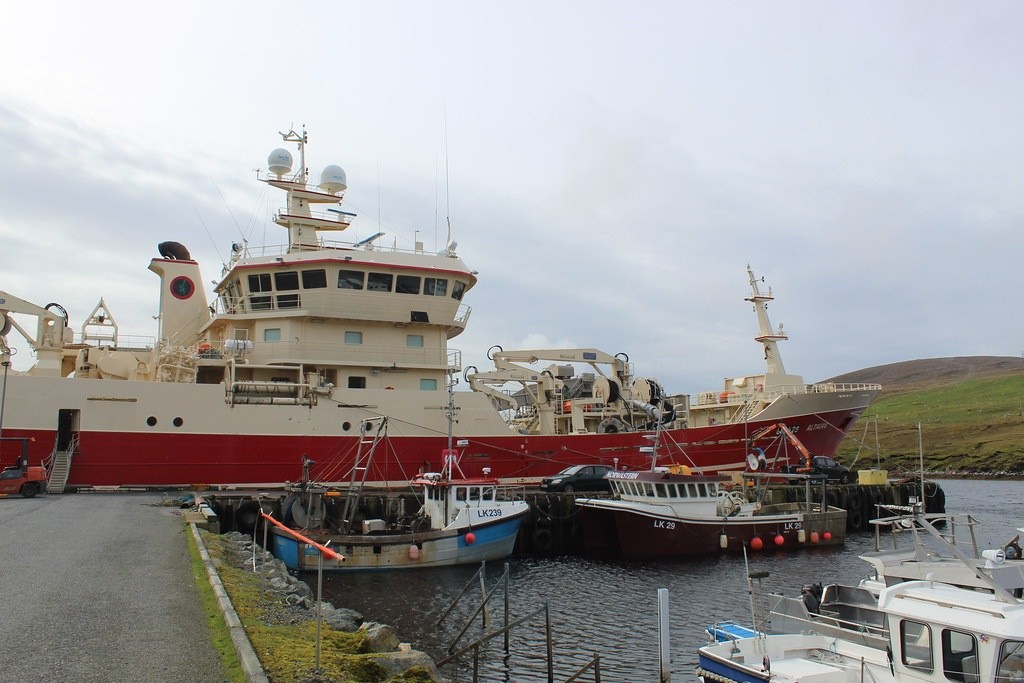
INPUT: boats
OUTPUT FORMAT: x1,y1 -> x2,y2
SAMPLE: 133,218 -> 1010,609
270,365 -> 531,571
0,119 -> 884,492
572,386 -> 848,556
694,420 -> 1024,682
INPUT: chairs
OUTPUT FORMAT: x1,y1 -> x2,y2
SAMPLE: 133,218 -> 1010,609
4,456 -> 21,471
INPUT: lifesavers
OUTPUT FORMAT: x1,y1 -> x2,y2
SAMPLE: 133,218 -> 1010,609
847,492 -> 865,530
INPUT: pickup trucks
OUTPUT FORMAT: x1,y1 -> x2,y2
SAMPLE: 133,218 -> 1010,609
778,455 -> 859,484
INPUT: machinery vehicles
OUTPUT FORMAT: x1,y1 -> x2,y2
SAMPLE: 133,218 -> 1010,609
749,422 -> 828,484
0,435 -> 49,499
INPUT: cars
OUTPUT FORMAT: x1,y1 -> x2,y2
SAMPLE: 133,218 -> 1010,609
539,463 -> 621,493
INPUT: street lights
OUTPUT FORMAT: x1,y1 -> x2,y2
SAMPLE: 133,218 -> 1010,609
0,350 -> 12,429
